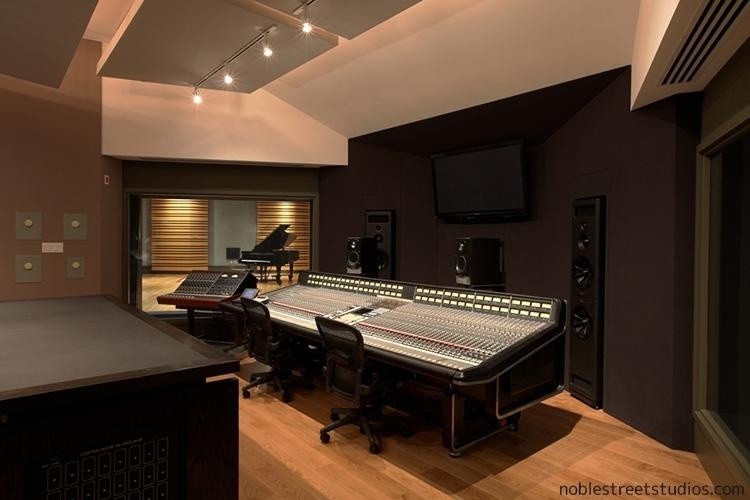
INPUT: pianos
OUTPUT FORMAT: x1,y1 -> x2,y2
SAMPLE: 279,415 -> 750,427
238,225 -> 299,285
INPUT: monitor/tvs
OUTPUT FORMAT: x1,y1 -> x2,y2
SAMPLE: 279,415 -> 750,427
430,137 -> 533,224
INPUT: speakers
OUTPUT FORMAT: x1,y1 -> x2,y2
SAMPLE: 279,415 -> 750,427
455,238 -> 504,286
366,211 -> 395,280
345,236 -> 378,278
569,197 -> 605,410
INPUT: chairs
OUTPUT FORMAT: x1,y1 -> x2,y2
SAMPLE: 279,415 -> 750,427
314,315 -> 415,455
239,296 -> 316,403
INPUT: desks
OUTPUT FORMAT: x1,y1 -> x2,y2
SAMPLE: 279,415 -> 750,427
1,293 -> 241,498
